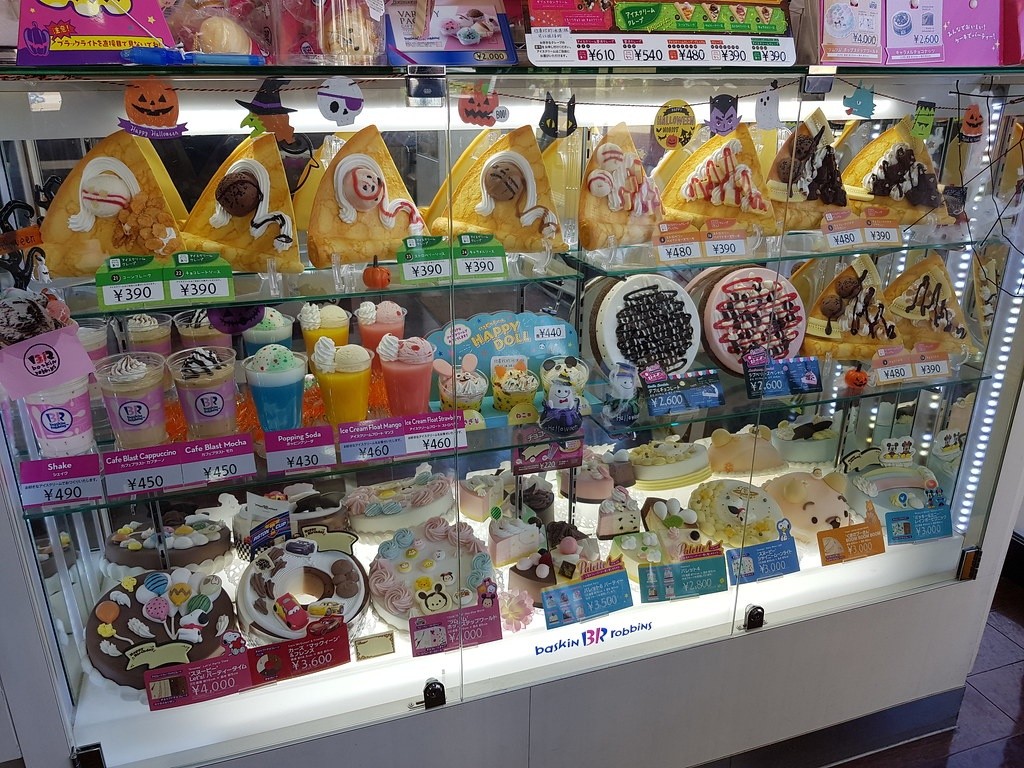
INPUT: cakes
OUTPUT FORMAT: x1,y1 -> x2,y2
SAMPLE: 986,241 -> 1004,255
80,411 -> 947,707
891,11 -> 912,36
696,264 -> 806,378
893,391 -> 976,507
590,274 -> 702,386
823,3 -> 855,38
33,530 -> 77,597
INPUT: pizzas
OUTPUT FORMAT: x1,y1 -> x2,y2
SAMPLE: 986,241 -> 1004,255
674,1 -> 773,25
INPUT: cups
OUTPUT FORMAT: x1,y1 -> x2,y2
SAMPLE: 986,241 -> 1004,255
173,309 -> 233,349
94,351 -> 165,451
354,304 -> 407,369
243,314 -> 295,356
311,347 -> 374,426
126,313 -> 172,357
77,317 -> 108,398
376,339 -> 437,417
298,309 -> 352,372
540,355 -> 589,408
166,346 -> 237,440
491,370 -> 539,411
22,374 -> 94,458
440,365 -> 490,412
240,353 -> 309,432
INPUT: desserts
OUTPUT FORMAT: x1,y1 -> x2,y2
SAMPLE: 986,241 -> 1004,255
30,108 -> 960,286
791,252 -> 979,363
441,9 -> 501,45
0,279 -> 591,464
950,106 -> 1024,351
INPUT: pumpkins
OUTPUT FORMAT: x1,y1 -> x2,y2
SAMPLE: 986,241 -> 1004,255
362,255 -> 392,289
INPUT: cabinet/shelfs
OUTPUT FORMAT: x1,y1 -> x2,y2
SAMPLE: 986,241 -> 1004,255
0,65 -> 1024,768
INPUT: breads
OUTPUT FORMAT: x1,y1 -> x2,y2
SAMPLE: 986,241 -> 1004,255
160,0 -> 377,56
